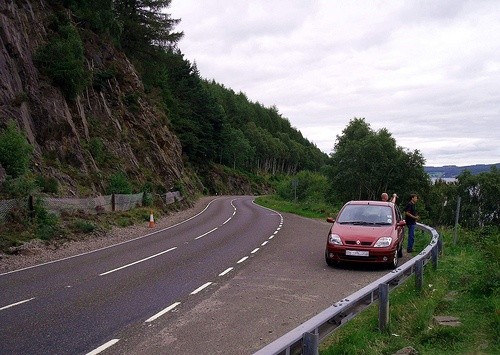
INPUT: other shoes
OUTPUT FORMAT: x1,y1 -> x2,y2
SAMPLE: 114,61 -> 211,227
407,249 -> 417,252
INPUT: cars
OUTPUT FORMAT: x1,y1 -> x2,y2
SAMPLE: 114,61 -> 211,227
325,199 -> 407,270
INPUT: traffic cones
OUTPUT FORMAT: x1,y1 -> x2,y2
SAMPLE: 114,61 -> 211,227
148,209 -> 156,229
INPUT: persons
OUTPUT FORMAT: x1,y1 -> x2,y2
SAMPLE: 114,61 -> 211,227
405,194 -> 420,252
381,193 -> 397,203
